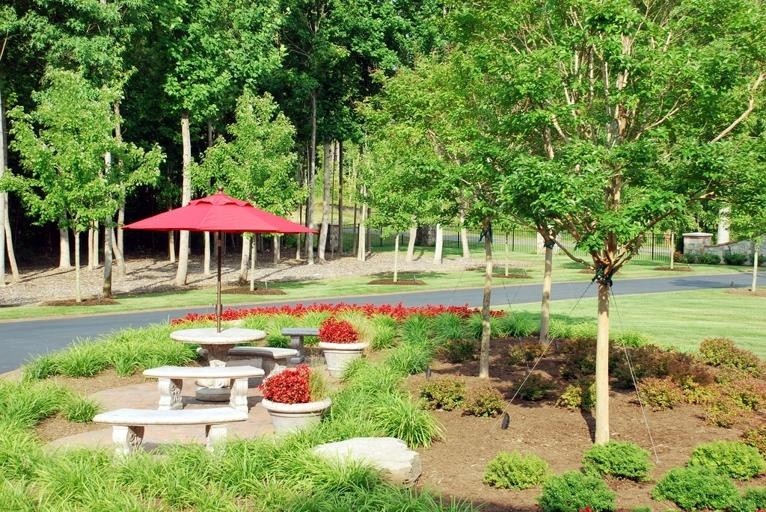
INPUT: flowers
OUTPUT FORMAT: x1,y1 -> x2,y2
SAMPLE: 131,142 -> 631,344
317,314 -> 361,344
255,362 -> 329,403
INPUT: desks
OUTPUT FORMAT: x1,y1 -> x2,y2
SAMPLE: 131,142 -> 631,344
170,325 -> 267,388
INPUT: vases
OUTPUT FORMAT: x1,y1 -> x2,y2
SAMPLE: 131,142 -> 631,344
259,398 -> 330,444
319,341 -> 366,380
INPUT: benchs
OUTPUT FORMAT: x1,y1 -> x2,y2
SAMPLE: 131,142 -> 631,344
88,345 -> 303,458
280,323 -> 320,361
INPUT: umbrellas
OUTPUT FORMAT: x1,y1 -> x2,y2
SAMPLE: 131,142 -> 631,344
122,187 -> 320,332
